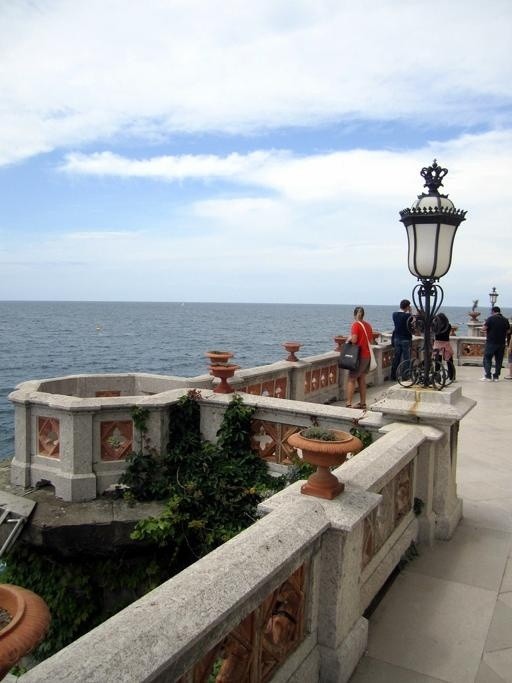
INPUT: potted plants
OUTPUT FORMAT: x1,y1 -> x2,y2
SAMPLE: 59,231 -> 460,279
285,425 -> 364,501
204,350 -> 241,394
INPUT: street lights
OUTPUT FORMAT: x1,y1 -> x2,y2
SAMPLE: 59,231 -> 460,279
488,286 -> 499,307
396,157 -> 468,389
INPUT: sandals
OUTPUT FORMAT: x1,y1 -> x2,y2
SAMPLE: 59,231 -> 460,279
354,403 -> 367,409
346,404 -> 352,408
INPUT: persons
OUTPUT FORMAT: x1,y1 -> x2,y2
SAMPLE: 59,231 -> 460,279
344,306 -> 374,409
429,312 -> 457,381
391,300 -> 413,381
481,306 -> 511,382
503,330 -> 512,380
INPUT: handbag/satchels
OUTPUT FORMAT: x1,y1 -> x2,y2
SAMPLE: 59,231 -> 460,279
368,344 -> 377,372
338,342 -> 361,372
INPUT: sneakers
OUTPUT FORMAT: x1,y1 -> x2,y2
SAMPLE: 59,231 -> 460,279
480,377 -> 499,382
